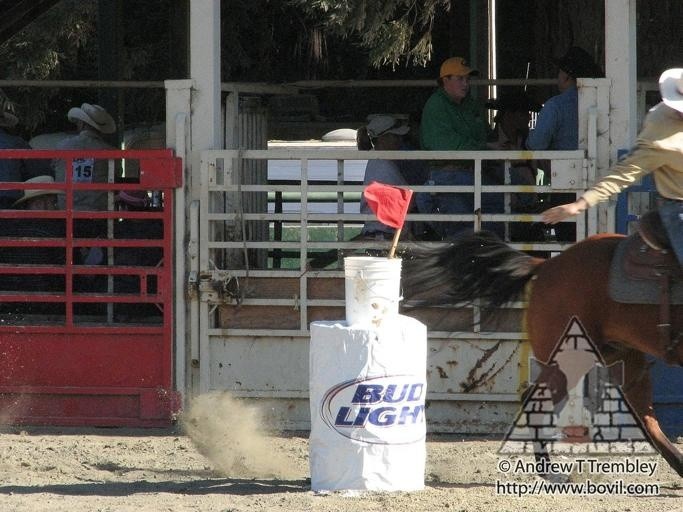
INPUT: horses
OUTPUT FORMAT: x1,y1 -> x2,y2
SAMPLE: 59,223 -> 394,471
400,231 -> 683,483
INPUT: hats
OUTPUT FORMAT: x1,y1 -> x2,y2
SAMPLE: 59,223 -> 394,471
365,114 -> 409,139
11,174 -> 68,208
657,66 -> 683,113
439,56 -> 480,78
67,101 -> 117,136
0,106 -> 21,130
552,46 -> 592,79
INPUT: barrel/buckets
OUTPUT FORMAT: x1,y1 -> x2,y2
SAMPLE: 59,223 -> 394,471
343,255 -> 403,328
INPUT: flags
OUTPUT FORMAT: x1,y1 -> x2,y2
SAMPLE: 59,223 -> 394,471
361,180 -> 412,232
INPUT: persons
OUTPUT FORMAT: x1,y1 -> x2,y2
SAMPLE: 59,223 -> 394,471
354,112 -> 424,238
0,107 -> 53,210
415,57 -> 527,243
76,175 -> 164,322
519,47 -> 606,240
46,101 -> 125,257
480,87 -> 553,226
0,172 -> 67,317
539,66 -> 683,283
